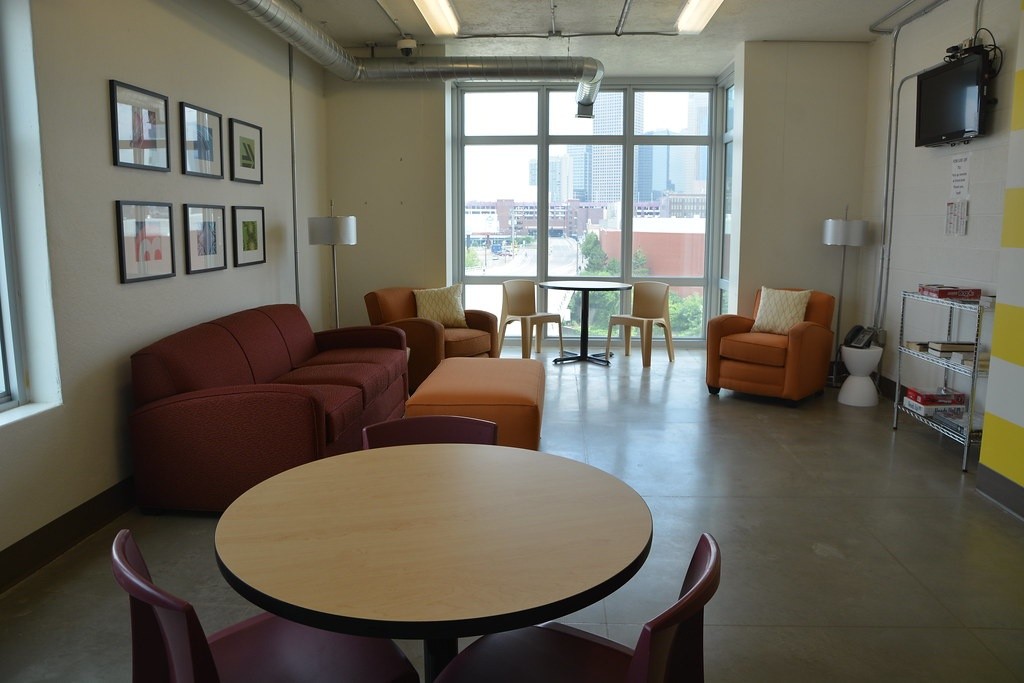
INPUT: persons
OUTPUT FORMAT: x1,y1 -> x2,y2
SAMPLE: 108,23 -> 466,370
126,110 -> 156,151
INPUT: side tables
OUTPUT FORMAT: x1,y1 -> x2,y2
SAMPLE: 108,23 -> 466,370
837,343 -> 883,406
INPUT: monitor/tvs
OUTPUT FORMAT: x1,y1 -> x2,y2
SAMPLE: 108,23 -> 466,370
913,49 -> 991,148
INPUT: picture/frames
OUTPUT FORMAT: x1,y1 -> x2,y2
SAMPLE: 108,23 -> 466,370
183,203 -> 227,274
179,102 -> 225,180
109,78 -> 172,172
230,206 -> 267,267
229,118 -> 263,185
115,200 -> 176,283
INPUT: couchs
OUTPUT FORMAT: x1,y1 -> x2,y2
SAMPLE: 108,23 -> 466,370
362,286 -> 498,396
128,304 -> 407,514
706,286 -> 835,404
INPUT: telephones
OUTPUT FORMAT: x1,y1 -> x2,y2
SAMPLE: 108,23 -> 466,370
844,325 -> 877,349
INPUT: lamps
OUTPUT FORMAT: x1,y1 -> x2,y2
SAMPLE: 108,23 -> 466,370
821,218 -> 869,388
307,200 -> 356,328
414,0 -> 460,40
674,0 -> 724,36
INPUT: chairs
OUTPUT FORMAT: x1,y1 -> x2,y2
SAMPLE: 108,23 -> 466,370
110,525 -> 420,683
605,281 -> 675,368
358,417 -> 498,450
499,278 -> 563,359
442,534 -> 721,683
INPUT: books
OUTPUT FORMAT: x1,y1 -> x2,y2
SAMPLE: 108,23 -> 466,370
905,340 -> 990,369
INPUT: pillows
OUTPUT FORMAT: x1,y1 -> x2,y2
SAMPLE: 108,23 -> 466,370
413,284 -> 467,328
749,288 -> 812,334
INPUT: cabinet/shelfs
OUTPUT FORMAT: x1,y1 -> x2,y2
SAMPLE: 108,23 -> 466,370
893,291 -> 994,475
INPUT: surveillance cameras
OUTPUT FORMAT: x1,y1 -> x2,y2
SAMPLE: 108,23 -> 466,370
396,39 -> 416,57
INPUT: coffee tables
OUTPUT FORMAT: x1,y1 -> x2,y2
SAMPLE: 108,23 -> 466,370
405,357 -> 547,451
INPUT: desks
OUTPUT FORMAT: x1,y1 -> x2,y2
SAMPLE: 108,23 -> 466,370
539,281 -> 633,366
213,442 -> 653,683
